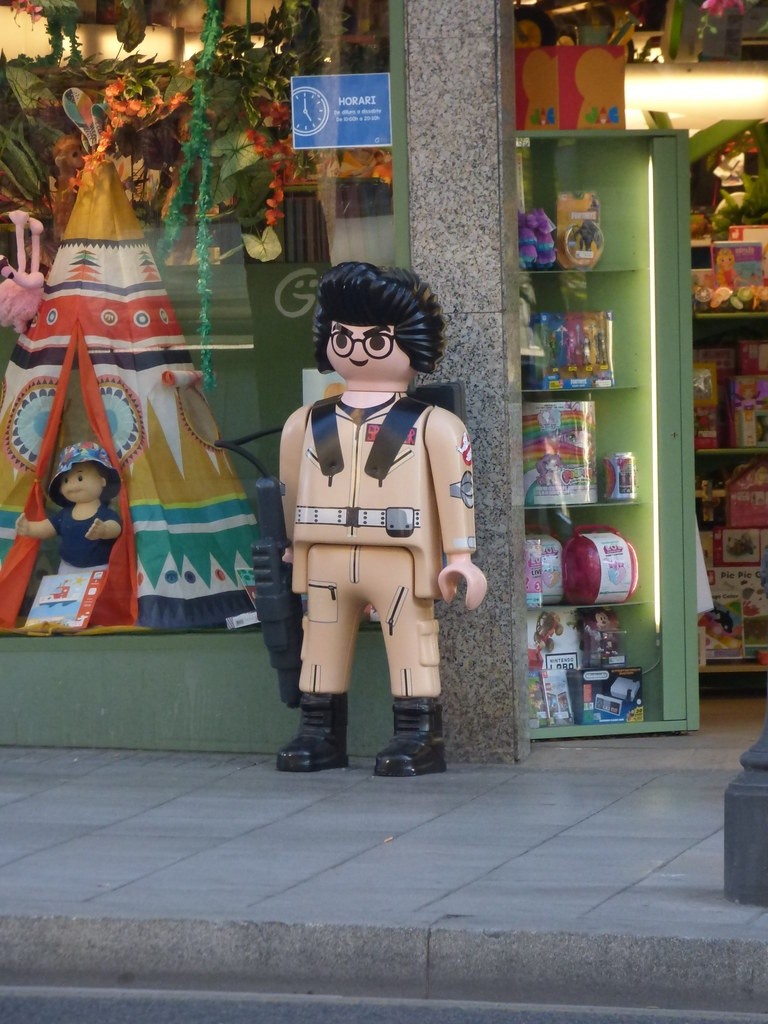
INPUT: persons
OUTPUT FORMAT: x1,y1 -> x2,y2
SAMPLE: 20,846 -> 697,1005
274,263 -> 486,776
14,441 -> 123,574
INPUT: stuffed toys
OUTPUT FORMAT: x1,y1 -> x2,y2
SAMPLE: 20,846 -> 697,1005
0,210 -> 44,334
50,136 -> 86,237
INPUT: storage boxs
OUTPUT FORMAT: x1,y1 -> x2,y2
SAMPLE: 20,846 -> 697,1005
540,668 -> 575,726
527,669 -> 549,728
527,310 -> 617,388
566,666 -> 644,724
692,339 -> 768,660
711,241 -> 761,294
603,630 -> 628,668
729,225 -> 768,286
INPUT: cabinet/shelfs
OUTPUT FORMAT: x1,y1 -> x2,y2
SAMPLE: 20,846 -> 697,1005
692,311 -> 768,672
518,127 -> 702,740
